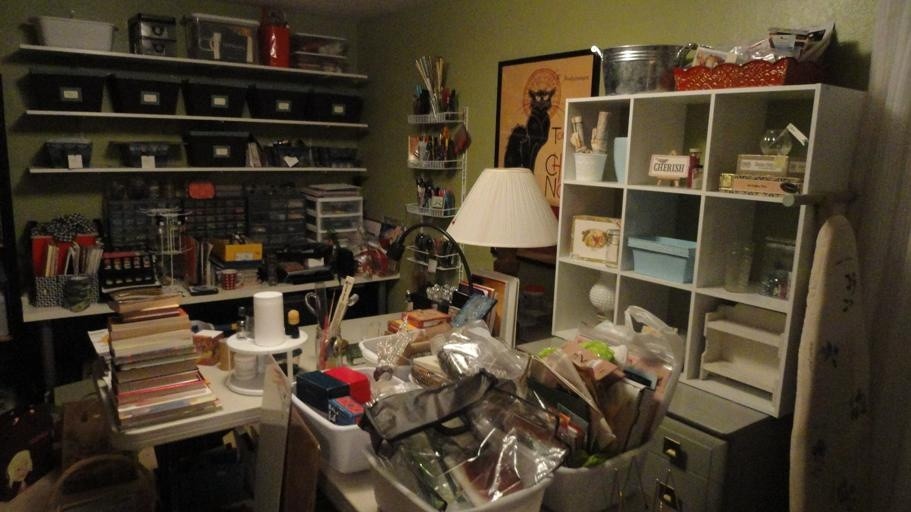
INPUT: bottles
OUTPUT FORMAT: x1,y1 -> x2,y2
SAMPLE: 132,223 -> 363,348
314,326 -> 343,370
687,148 -> 704,190
237,307 -> 254,339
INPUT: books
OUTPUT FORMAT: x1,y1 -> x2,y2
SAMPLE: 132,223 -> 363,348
83,288 -> 226,434
41,242 -> 105,278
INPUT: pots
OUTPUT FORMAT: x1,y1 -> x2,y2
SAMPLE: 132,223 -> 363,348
591,43 -> 699,96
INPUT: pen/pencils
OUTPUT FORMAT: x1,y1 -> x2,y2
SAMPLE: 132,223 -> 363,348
415,234 -> 458,267
444,87 -> 457,120
407,126 -> 455,170
416,178 -> 455,218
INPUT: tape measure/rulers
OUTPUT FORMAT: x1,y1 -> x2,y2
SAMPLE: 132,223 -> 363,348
313,275 -> 356,335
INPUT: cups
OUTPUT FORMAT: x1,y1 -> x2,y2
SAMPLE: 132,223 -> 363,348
221,269 -> 244,291
723,240 -> 755,292
605,229 -> 620,270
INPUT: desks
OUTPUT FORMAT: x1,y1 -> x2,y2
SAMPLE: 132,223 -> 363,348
89,352 -> 291,511
19,267 -> 401,392
273,310 -> 402,511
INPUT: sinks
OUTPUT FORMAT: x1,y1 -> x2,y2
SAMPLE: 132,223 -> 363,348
339,311 -> 406,342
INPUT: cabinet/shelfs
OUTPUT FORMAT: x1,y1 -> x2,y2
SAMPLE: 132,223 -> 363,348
518,335 -> 791,512
103,185 -> 365,252
551,81 -> 868,424
698,302 -> 787,405
407,105 -> 469,286
17,41 -> 371,175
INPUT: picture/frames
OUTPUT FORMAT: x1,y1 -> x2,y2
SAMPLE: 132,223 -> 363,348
494,49 -> 600,209
568,214 -> 621,264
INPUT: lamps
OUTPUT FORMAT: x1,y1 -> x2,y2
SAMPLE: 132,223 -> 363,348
443,166 -> 557,277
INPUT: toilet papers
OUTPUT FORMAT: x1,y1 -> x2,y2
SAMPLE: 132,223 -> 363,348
252,291 -> 285,348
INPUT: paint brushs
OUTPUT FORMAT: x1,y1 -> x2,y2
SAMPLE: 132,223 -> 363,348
415,54 -> 444,123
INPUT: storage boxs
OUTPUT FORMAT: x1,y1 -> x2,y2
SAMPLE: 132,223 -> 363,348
478,404 -> 655,512
360,416 -> 558,512
292,365 -> 416,477
358,331 -> 403,361
292,33 -> 348,74
127,12 -> 260,64
